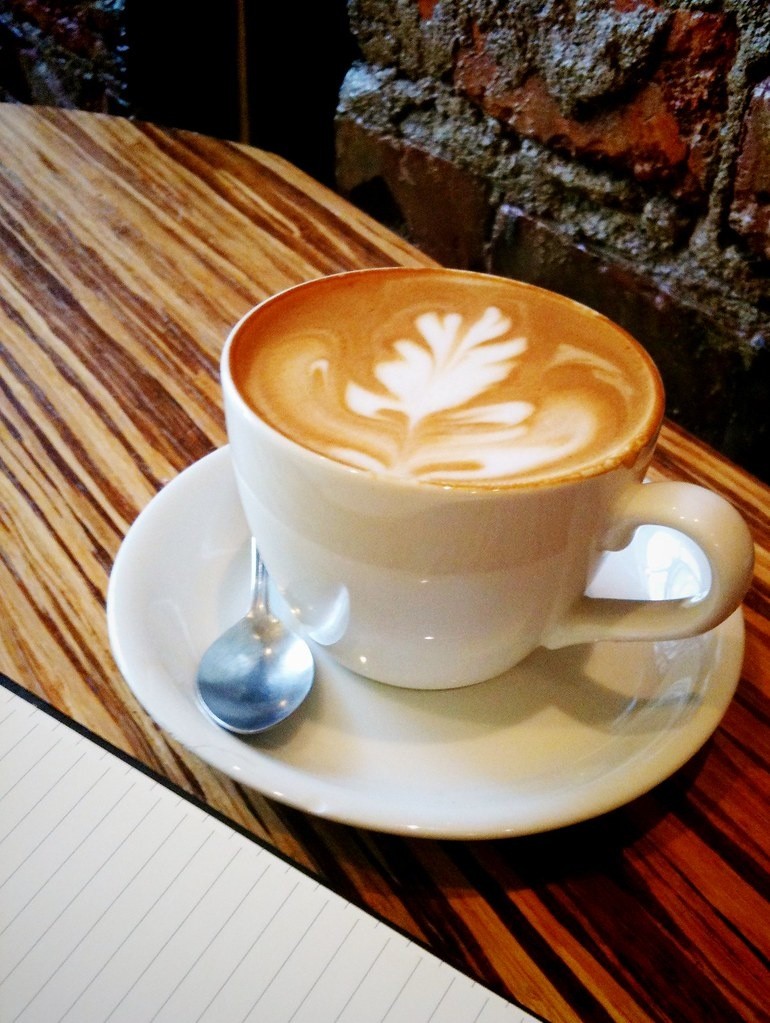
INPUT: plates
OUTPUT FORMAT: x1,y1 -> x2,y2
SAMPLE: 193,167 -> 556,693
106,444 -> 745,840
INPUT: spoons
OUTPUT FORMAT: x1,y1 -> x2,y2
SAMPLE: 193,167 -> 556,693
194,530 -> 313,736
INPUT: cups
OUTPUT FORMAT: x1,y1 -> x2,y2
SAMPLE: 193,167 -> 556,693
220,266 -> 757,690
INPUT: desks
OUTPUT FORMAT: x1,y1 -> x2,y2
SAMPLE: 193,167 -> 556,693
0,104 -> 770,1023
